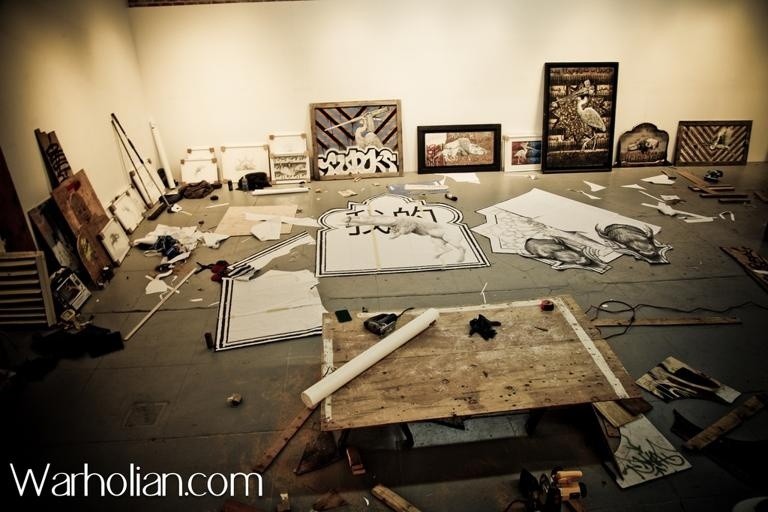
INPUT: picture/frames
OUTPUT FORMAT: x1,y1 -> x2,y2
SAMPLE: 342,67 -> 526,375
269,135 -> 307,156
309,99 -> 403,181
108,190 -> 146,236
221,145 -> 270,182
129,158 -> 167,209
96,216 -> 133,268
180,159 -> 218,185
188,149 -> 215,160
542,61 -> 619,174
115,183 -> 149,211
676,121 -> 753,166
504,136 -> 542,172
269,152 -> 311,185
417,124 -> 501,174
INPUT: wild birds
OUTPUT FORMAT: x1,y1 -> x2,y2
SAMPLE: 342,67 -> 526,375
514,142 -> 538,165
323,106 -> 389,151
567,91 -> 607,156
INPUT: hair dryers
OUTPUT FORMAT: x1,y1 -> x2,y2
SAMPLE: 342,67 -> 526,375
301,309 -> 442,411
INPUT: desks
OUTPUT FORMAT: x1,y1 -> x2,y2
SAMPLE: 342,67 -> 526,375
319,296 -> 645,480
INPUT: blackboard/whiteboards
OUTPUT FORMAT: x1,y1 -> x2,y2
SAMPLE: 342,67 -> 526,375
181,133 -> 309,187
98,159 -> 167,267
